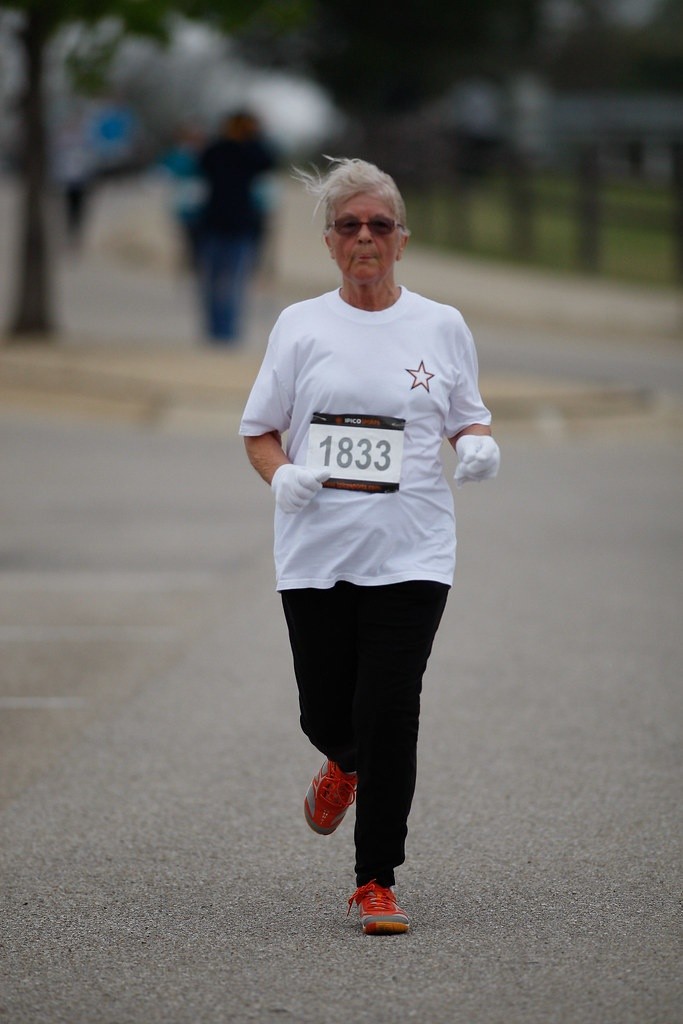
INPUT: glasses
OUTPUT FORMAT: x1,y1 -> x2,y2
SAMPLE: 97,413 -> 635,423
328,215 -> 403,236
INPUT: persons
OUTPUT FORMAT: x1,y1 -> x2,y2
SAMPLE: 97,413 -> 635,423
238,153 -> 500,935
181,110 -> 286,350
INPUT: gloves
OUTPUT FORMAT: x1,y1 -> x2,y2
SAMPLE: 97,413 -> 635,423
270,463 -> 331,516
456,434 -> 499,485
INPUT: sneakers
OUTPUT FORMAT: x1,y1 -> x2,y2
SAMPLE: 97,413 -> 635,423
305,757 -> 357,836
356,884 -> 412,935
